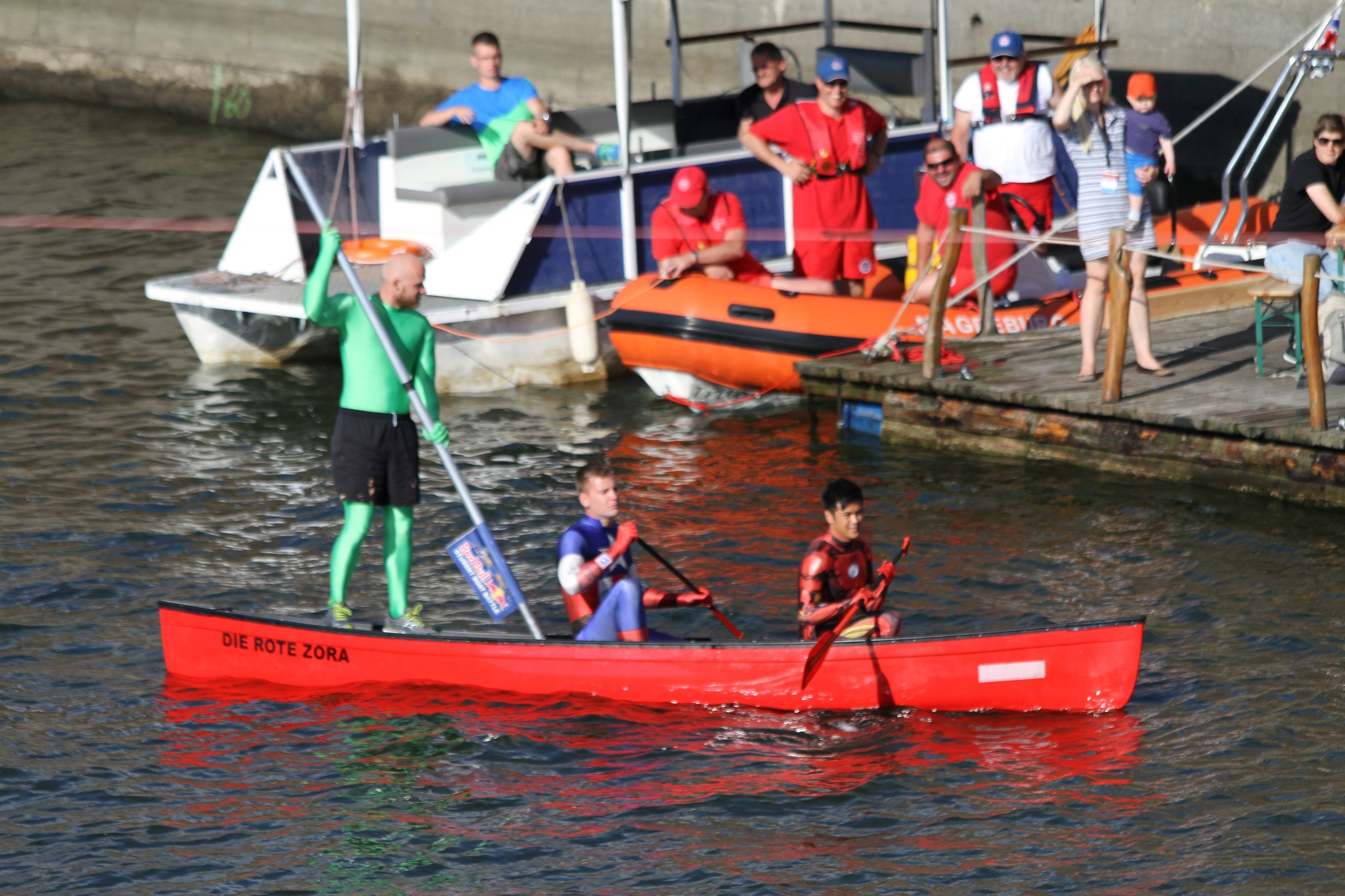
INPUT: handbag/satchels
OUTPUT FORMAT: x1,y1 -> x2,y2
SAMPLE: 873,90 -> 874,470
1150,180 -> 1174,215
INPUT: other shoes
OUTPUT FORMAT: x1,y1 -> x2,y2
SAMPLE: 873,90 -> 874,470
1324,363 -> 1345,385
1283,347 -> 1298,364
598,144 -> 619,167
1122,216 -> 1141,232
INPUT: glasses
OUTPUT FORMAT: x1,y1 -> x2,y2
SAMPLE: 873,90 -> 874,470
1318,137 -> 1345,147
928,157 -> 953,168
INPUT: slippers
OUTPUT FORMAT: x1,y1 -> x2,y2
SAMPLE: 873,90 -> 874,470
1136,363 -> 1172,377
1077,374 -> 1095,383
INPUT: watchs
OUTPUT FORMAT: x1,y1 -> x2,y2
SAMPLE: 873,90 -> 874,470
541,113 -> 552,124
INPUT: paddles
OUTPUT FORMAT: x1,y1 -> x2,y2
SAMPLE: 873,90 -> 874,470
637,535 -> 750,640
801,535 -> 911,689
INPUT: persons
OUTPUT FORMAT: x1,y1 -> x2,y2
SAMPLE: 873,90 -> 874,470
305,219 -> 449,639
651,166 -> 837,295
1122,72 -> 1176,231
420,32 -> 629,180
747,51 -> 887,297
555,463 -> 715,643
1264,114 -> 1345,366
902,138 -> 1019,303
797,477 -> 903,641
950,31 -> 1067,253
1053,56 -> 1173,382
737,42 -> 819,149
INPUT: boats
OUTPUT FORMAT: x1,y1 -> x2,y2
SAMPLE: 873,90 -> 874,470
155,600 -> 1148,714
138,0 -> 1111,399
608,195 -> 1278,414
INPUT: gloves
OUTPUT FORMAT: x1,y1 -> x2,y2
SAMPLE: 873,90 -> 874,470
581,520 -> 637,587
845,590 -> 868,611
641,585 -> 713,609
877,561 -> 896,583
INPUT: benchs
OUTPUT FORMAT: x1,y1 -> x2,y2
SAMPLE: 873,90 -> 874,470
1247,277 -> 1304,381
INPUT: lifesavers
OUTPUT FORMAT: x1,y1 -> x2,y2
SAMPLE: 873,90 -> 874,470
340,238 -> 434,266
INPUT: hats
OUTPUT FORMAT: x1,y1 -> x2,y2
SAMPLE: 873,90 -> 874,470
1127,73 -> 1156,99
817,58 -> 849,83
989,32 -> 1023,58
670,166 -> 707,208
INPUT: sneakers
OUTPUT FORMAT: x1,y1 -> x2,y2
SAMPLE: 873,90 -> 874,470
325,599 -> 354,630
382,602 -> 436,636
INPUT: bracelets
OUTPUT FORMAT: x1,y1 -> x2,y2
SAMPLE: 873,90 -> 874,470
691,250 -> 699,265
